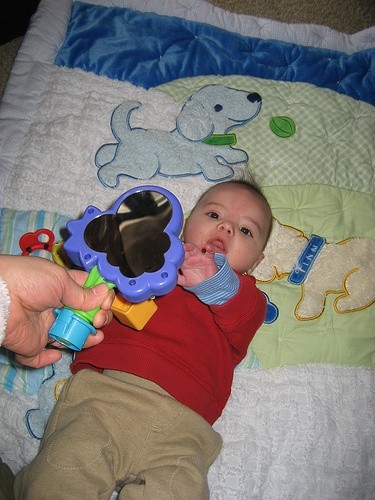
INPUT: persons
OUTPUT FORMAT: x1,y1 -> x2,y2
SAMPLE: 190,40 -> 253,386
1,252 -> 114,369
13,160 -> 272,500
